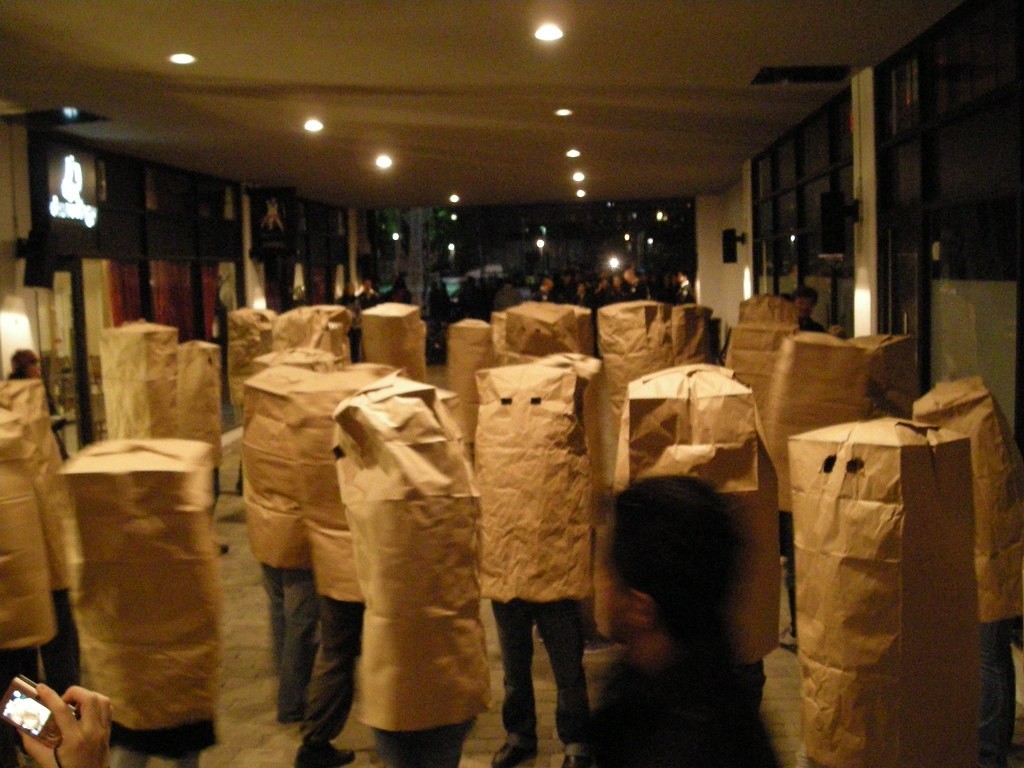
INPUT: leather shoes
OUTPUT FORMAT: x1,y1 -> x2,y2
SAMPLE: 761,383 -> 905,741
491,742 -> 537,768
559,754 -> 586,768
278,711 -> 303,723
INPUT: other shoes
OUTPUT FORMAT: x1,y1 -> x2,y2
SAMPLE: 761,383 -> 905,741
295,742 -> 356,768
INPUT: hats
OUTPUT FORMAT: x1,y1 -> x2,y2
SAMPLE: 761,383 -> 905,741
12,350 -> 40,371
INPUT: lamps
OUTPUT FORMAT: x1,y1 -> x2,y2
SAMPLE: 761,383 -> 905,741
722,228 -> 746,263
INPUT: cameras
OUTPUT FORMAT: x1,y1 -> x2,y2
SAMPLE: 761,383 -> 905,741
0,674 -> 79,749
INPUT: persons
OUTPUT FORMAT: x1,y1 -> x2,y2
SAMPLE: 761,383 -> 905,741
0,267 -> 1024,768
